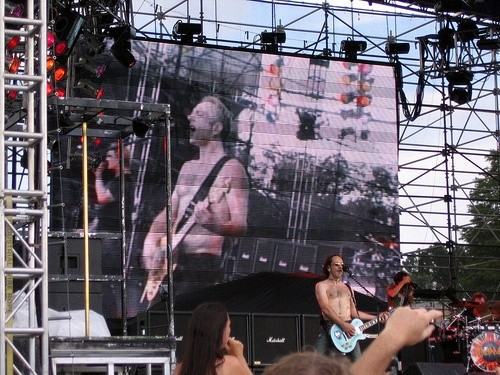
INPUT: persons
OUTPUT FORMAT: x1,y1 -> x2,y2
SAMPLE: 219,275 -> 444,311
262,307 -> 443,375
167,303 -> 252,374
386,271 -> 414,307
312,255 -> 390,362
464,293 -> 487,320
77,96 -> 250,311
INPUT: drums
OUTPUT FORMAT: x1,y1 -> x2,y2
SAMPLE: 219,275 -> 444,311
462,325 -> 495,337
470,332 -> 500,372
439,327 -> 457,342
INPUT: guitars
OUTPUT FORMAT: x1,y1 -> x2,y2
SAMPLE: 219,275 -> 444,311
146,198 -> 211,302
330,311 -> 393,353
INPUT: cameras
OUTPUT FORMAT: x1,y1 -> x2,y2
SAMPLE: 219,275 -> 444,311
404,281 -> 418,289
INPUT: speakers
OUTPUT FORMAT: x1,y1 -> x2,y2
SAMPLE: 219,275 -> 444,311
403,362 -> 467,375
137,310 -> 320,366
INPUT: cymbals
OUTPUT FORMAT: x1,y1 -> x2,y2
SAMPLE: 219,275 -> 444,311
449,300 -> 480,308
478,301 -> 500,319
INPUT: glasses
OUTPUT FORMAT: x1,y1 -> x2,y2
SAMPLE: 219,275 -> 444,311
331,262 -> 345,269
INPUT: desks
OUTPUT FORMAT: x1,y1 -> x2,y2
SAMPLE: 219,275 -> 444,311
50,336 -> 183,375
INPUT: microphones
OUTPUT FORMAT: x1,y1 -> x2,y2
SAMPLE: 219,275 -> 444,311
342,266 -> 354,277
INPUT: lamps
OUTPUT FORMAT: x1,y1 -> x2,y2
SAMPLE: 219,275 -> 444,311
110,45 -> 137,68
5,4 -> 67,99
175,23 -> 202,34
77,52 -> 105,79
385,43 -> 410,54
75,80 -> 106,100
267,58 -> 376,142
259,32 -> 286,44
340,39 -> 366,51
